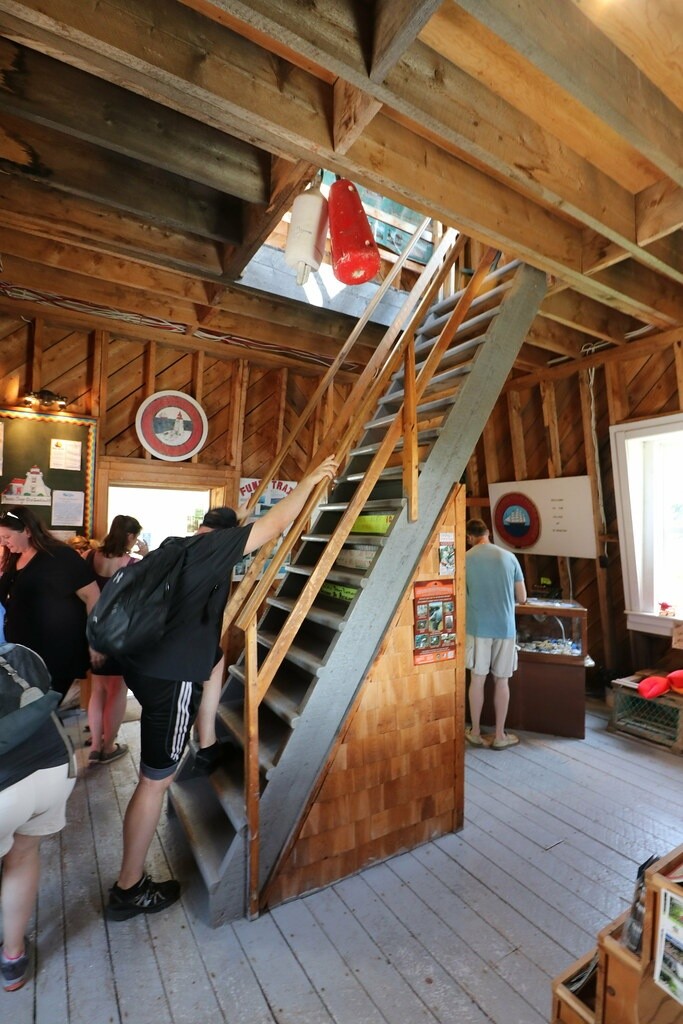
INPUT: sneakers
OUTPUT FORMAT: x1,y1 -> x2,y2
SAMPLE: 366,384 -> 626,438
191,740 -> 236,778
0,936 -> 32,991
104,871 -> 181,922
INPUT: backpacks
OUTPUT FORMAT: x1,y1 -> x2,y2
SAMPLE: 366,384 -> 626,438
81,535 -> 226,666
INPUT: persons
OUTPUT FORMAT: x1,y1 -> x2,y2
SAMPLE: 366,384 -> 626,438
0,454 -> 339,992
466,518 -> 527,750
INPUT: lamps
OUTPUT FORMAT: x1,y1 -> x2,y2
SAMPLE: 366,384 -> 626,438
25,389 -> 67,410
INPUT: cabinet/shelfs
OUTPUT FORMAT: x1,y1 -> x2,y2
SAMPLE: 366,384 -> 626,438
475,599 -> 589,739
552,843 -> 683,1024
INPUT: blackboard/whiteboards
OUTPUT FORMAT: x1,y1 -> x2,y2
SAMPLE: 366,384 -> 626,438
0,404 -> 102,546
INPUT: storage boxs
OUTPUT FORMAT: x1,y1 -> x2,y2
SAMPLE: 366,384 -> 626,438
606,668 -> 683,757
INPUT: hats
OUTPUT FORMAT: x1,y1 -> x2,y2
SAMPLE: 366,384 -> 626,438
201,506 -> 238,533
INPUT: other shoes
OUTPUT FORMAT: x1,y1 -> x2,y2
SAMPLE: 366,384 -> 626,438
463,722 -> 484,747
87,742 -> 129,765
490,730 -> 521,751
